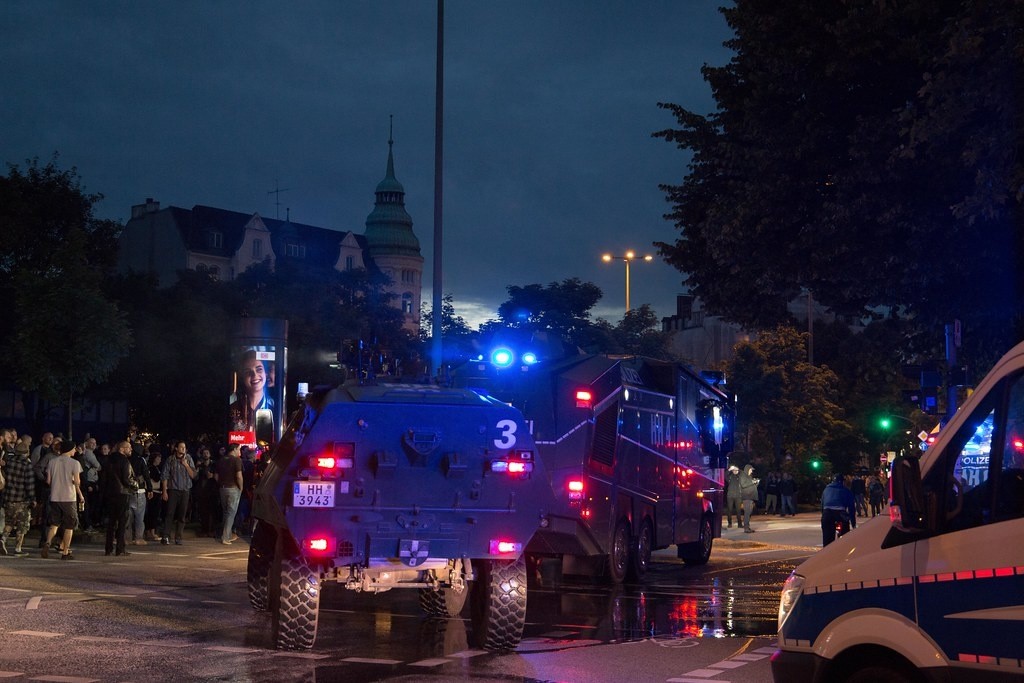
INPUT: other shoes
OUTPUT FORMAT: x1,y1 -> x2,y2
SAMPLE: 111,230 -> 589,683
13,550 -> 29,556
116,551 -> 132,556
105,552 -> 113,556
728,524 -> 732,527
161,536 -> 170,545
84,526 -> 99,533
58,549 -> 72,554
738,525 -> 744,528
148,535 -> 162,540
41,543 -> 50,559
124,539 -> 134,545
231,533 -> 238,541
135,539 -> 147,545
0,540 -> 7,555
61,553 -> 73,560
744,528 -> 754,533
176,538 -> 183,545
223,540 -> 231,544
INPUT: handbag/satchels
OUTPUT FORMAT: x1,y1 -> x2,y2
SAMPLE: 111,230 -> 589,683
144,466 -> 161,490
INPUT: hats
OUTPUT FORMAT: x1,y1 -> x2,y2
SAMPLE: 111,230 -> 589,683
15,442 -> 28,455
60,440 -> 75,453
834,473 -> 846,481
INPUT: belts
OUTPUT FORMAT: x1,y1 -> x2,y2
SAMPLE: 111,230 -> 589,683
221,485 -> 238,489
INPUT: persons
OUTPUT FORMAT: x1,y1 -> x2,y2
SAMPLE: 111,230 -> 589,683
232,350 -> 276,428
725,456 -> 890,547
0,425 -> 274,560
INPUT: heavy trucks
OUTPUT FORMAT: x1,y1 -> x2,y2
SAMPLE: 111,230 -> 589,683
245,346 -> 742,656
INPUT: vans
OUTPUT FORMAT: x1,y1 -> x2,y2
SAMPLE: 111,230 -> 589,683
767,342 -> 1024,683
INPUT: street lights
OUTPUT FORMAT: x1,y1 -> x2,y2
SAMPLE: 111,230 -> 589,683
602,249 -> 654,317
